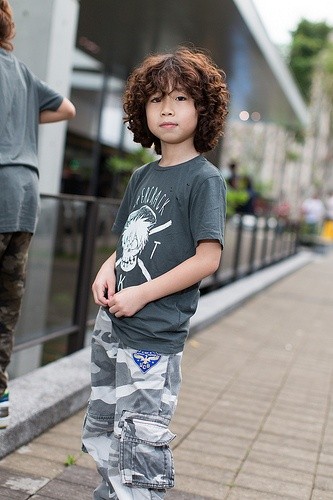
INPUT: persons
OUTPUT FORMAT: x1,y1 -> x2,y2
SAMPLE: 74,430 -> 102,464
222,160 -> 333,232
77,46 -> 231,500
0,1 -> 77,432
